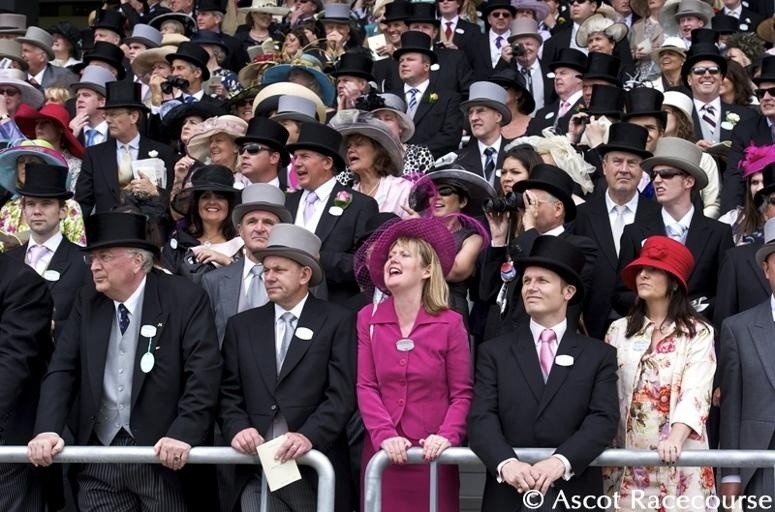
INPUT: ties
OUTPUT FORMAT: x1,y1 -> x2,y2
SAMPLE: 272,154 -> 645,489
118,304 -> 130,335
445,22 -> 452,40
614,204 -> 628,254
26,243 -> 47,273
273,313 -> 296,444
540,328 -> 556,376
245,267 -> 266,309
670,222 -> 686,245
300,194 -> 318,228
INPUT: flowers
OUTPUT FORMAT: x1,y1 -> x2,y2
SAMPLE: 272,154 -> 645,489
332,188 -> 355,213
724,113 -> 742,129
420,91 -> 440,106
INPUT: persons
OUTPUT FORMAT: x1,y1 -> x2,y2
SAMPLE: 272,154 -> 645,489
0,0 -> 775,511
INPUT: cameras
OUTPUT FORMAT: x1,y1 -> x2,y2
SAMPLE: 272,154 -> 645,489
511,43 -> 527,56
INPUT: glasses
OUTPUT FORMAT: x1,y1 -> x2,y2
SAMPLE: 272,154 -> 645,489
427,187 -> 463,198
650,169 -> 684,178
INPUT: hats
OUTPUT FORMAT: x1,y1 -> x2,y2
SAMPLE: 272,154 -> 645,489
597,122 -> 654,165
251,223 -> 323,287
737,143 -> 775,270
512,236 -> 587,303
620,234 -> 696,295
409,163 -> 497,216
0,0 -> 441,174
171,164 -> 241,216
458,0 -> 775,129
15,162 -> 74,200
640,136 -> 708,190
367,217 -> 457,296
231,184 -> 293,227
512,163 -> 577,223
77,212 -> 160,256
